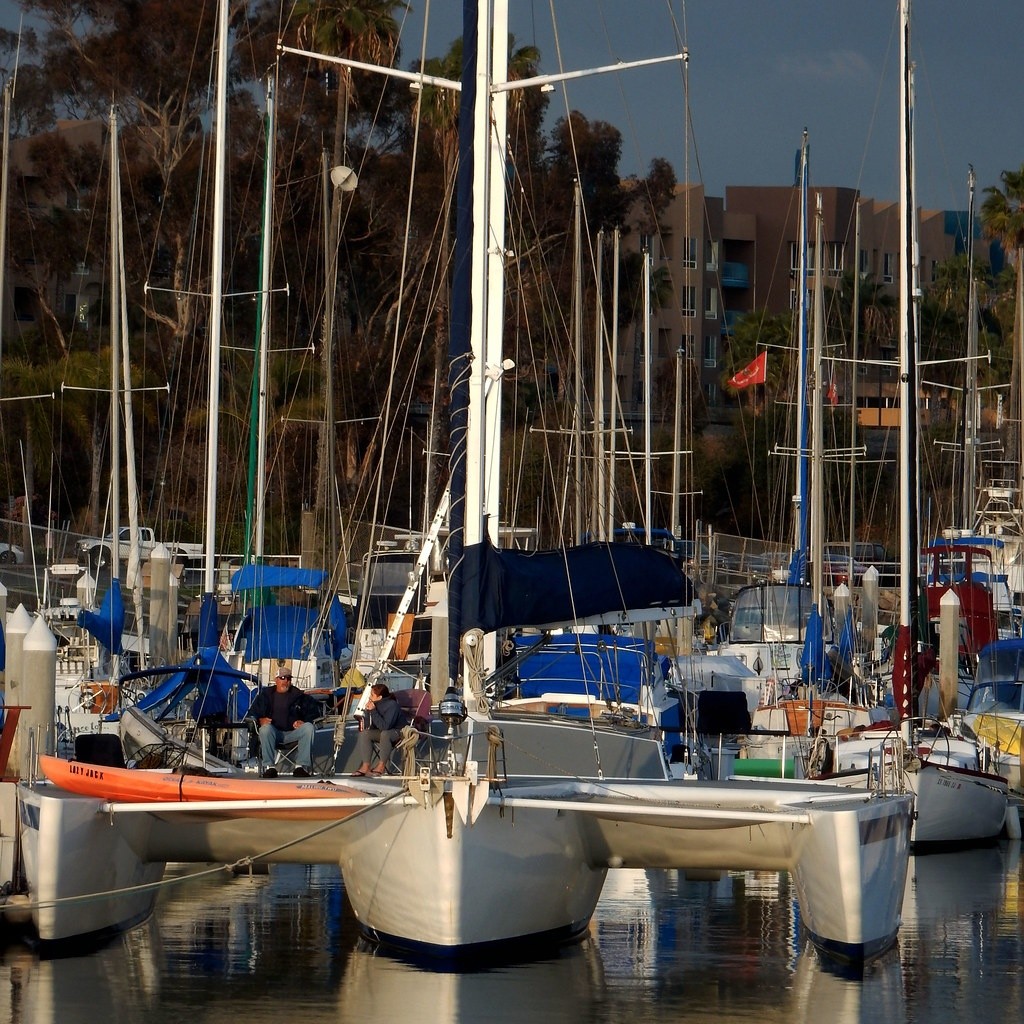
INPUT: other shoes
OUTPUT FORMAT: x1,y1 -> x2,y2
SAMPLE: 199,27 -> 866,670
293,767 -> 310,777
263,768 -> 278,778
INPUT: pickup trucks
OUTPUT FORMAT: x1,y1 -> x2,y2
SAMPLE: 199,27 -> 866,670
75,525 -> 204,571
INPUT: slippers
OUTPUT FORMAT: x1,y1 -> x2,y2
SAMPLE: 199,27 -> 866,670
366,768 -> 385,777
351,770 -> 370,777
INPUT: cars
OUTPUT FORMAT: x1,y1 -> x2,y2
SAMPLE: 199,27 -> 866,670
809,554 -> 870,586
719,552 -> 771,582
0,543 -> 26,565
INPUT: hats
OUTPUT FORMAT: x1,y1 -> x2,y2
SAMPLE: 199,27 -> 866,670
276,668 -> 293,678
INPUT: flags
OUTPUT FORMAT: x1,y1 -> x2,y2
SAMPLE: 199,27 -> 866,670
726,350 -> 766,387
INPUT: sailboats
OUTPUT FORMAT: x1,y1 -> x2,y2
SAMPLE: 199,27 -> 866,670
0,0 -> 1024,966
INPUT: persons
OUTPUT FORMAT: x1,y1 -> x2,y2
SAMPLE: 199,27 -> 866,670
351,684 -> 406,777
249,667 -> 316,778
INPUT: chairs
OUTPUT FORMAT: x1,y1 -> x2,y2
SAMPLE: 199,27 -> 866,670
370,689 -> 439,773
247,716 -> 314,777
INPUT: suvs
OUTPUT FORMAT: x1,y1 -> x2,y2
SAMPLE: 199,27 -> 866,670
674,540 -> 727,581
811,543 -> 888,567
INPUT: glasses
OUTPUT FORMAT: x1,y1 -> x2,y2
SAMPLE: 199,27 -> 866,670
278,676 -> 291,680
